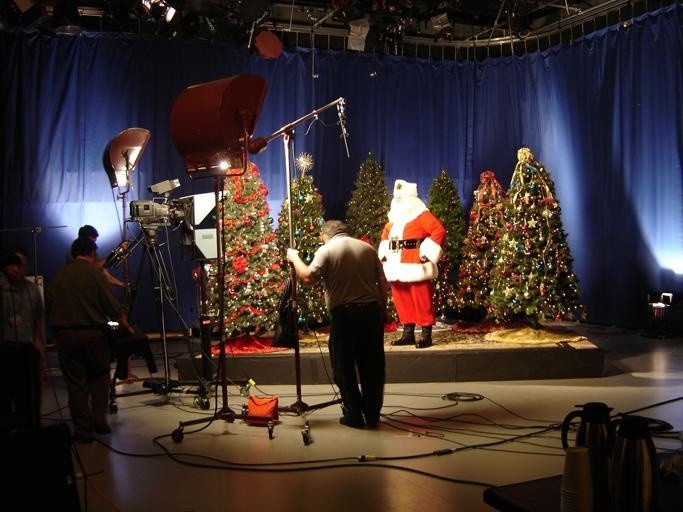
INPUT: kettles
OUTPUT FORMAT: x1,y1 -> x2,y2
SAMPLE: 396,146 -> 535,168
561,401 -> 659,512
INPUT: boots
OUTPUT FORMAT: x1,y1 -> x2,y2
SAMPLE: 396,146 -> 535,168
416,326 -> 432,348
391,324 -> 415,345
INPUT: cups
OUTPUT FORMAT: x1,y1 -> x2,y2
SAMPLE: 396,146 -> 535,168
560,447 -> 592,512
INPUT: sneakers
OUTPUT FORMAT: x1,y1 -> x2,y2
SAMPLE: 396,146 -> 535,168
340,418 -> 377,428
91,416 -> 110,434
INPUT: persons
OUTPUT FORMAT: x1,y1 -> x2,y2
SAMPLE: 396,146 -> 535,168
286,219 -> 389,430
0,246 -> 44,435
66,225 -> 131,288
43,237 -> 136,444
379,177 -> 447,348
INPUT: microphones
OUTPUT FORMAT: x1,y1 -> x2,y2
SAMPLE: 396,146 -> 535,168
338,119 -> 351,159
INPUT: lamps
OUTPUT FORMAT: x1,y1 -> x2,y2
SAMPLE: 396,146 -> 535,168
142,0 -> 176,23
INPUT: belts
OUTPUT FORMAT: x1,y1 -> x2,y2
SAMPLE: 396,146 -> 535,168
389,240 -> 423,250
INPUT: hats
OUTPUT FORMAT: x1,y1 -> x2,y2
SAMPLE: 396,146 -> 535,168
394,179 -> 417,196
